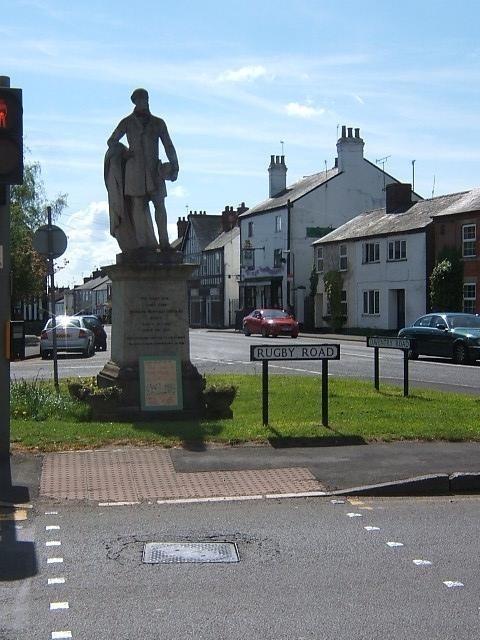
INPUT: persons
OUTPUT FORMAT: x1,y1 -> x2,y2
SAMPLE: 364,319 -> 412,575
105,88 -> 180,256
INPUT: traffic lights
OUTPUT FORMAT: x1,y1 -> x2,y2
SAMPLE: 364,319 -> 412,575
0,88 -> 23,186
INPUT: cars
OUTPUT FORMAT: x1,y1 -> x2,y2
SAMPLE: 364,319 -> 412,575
397,311 -> 480,365
38,314 -> 97,359
75,314 -> 109,351
241,307 -> 302,339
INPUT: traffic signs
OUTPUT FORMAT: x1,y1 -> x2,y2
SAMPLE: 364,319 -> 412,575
249,343 -> 340,361
366,336 -> 417,349
